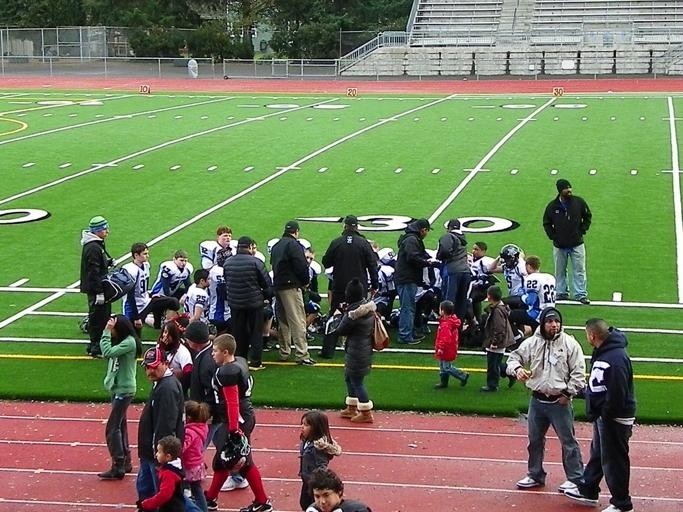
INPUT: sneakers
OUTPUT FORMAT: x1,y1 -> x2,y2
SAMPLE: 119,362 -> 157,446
517,478 -> 540,488
248,334 -> 315,371
581,298 -> 590,303
555,294 -> 568,299
482,386 -> 498,391
240,500 -> 273,511
601,503 -> 634,512
98,470 -> 125,480
508,375 -> 516,387
399,325 -> 430,345
558,481 -> 598,506
435,384 -> 447,387
220,475 -> 249,491
460,374 -> 469,386
206,500 -> 218,509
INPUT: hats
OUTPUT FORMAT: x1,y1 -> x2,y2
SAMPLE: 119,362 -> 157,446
285,222 -> 299,233
544,310 -> 561,323
345,278 -> 363,302
238,238 -> 252,247
448,219 -> 460,228
416,219 -> 430,231
89,216 -> 108,232
140,347 -> 165,367
557,180 -> 571,192
343,214 -> 356,226
185,321 -> 209,344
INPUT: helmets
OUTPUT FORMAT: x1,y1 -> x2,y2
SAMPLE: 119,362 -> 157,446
221,431 -> 252,473
501,244 -> 520,269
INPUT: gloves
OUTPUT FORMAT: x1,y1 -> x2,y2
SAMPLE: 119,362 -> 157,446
95,294 -> 105,305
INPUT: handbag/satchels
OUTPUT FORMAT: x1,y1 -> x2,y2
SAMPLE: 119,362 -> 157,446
373,318 -> 390,350
100,268 -> 135,303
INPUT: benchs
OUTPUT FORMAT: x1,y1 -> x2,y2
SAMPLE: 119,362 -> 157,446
408,1 -> 670,48
342,47 -> 682,75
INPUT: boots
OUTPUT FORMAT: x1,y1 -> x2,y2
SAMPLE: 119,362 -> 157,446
340,396 -> 358,417
351,400 -> 374,423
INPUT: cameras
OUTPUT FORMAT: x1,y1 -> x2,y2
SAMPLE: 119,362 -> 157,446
338,302 -> 349,313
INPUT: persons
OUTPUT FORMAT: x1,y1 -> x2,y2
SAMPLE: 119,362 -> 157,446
506,307 -> 586,492
564,318 -> 635,512
543,179 -> 592,305
